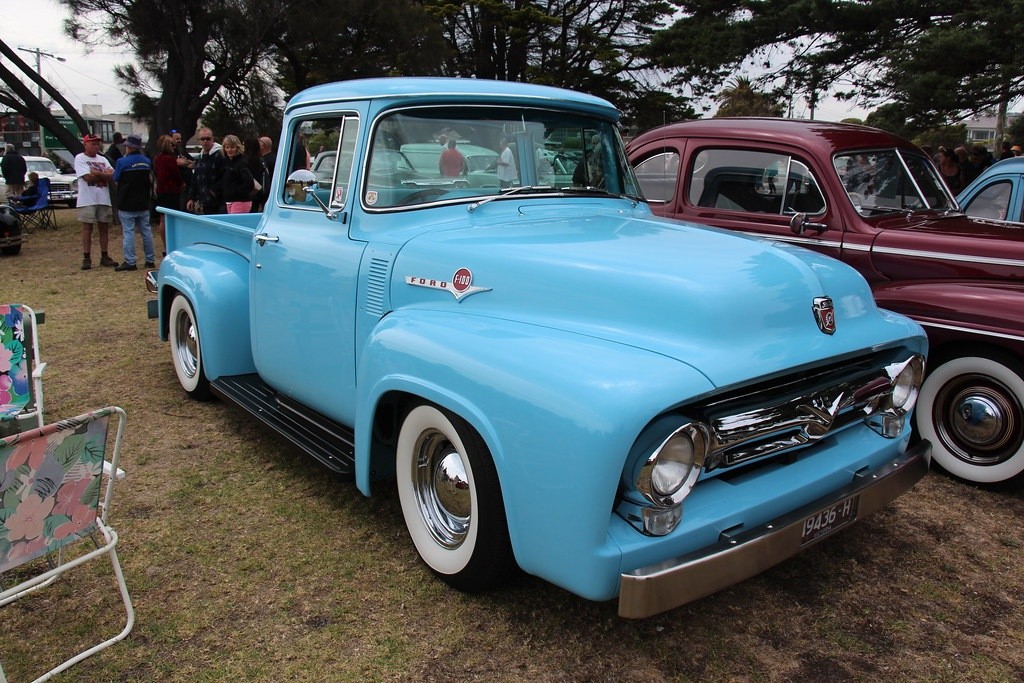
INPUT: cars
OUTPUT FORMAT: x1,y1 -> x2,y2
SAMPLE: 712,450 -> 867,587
394,143 -> 581,190
0,156 -> 80,209
622,118 -> 1024,494
950,153 -> 1024,222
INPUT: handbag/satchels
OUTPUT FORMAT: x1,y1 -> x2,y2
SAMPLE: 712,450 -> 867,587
248,178 -> 262,197
226,201 -> 252,213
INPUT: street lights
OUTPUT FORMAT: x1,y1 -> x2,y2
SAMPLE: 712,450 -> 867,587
35,49 -> 69,156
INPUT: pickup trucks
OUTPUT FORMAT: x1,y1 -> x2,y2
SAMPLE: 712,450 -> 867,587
144,77 -> 932,621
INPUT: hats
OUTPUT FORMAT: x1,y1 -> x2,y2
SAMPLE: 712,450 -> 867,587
7,143 -> 15,151
114,132 -> 126,145
123,135 -> 145,150
1011,145 -> 1023,154
83,134 -> 103,145
971,146 -> 993,154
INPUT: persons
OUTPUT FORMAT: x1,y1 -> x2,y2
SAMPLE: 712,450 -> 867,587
754,142 -> 1024,206
0,144 -> 40,227
74,134 -> 119,270
438,134 -> 604,189
105,127 -> 327,271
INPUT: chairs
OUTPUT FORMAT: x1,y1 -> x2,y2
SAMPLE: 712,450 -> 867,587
9,178 -> 57,233
0,304 -> 135,683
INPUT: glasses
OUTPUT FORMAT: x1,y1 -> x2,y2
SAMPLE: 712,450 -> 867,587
170,129 -> 177,133
200,137 -> 213,141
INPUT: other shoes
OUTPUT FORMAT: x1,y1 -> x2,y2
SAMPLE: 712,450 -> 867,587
82,258 -> 91,269
100,257 -> 119,267
115,262 -> 138,271
145,263 -> 155,268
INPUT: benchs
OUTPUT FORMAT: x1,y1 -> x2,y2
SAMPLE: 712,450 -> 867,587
638,176 -> 741,204
309,188 -> 450,206
